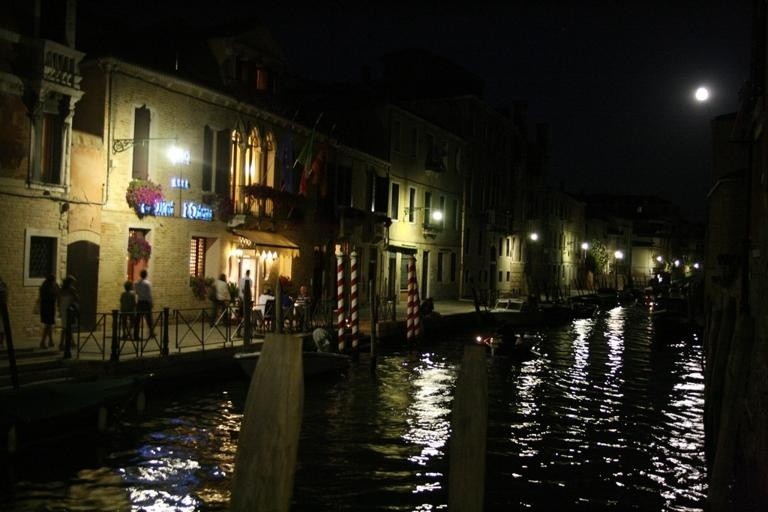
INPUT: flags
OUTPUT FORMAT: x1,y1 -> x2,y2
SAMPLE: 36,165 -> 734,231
294,127 -> 316,167
298,149 -> 322,196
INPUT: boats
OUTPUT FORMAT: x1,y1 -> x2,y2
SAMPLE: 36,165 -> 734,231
574,282 -> 684,317
232,349 -> 353,383
489,297 -> 573,325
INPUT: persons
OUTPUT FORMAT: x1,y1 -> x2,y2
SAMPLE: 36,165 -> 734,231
282,289 -> 293,309
207,273 -> 232,327
56,274 -> 81,350
37,273 -> 60,348
257,287 -> 276,320
239,269 -> 253,313
134,269 -> 158,341
118,279 -> 137,339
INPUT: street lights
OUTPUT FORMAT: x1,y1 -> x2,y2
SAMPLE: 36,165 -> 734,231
613,249 -> 623,290
